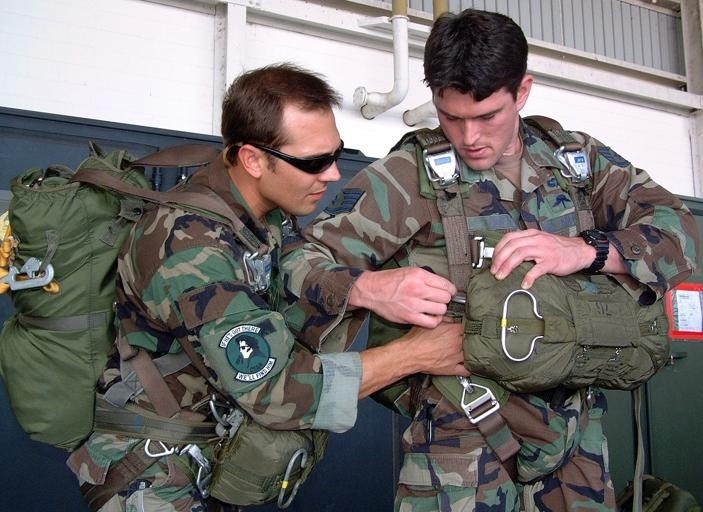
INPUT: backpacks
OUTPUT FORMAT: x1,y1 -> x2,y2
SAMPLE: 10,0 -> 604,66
0,141 -> 268,448
365,116 -> 596,417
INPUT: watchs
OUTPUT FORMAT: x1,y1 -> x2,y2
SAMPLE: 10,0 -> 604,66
576,229 -> 609,274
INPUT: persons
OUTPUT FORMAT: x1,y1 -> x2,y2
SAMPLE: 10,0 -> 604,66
66,64 -> 470,511
279,10 -> 699,512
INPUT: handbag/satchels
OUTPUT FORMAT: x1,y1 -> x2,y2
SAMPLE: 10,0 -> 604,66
463,259 -> 670,394
209,415 -> 330,505
616,474 -> 702,511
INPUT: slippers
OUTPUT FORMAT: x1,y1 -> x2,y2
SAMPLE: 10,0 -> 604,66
244,139 -> 344,174
